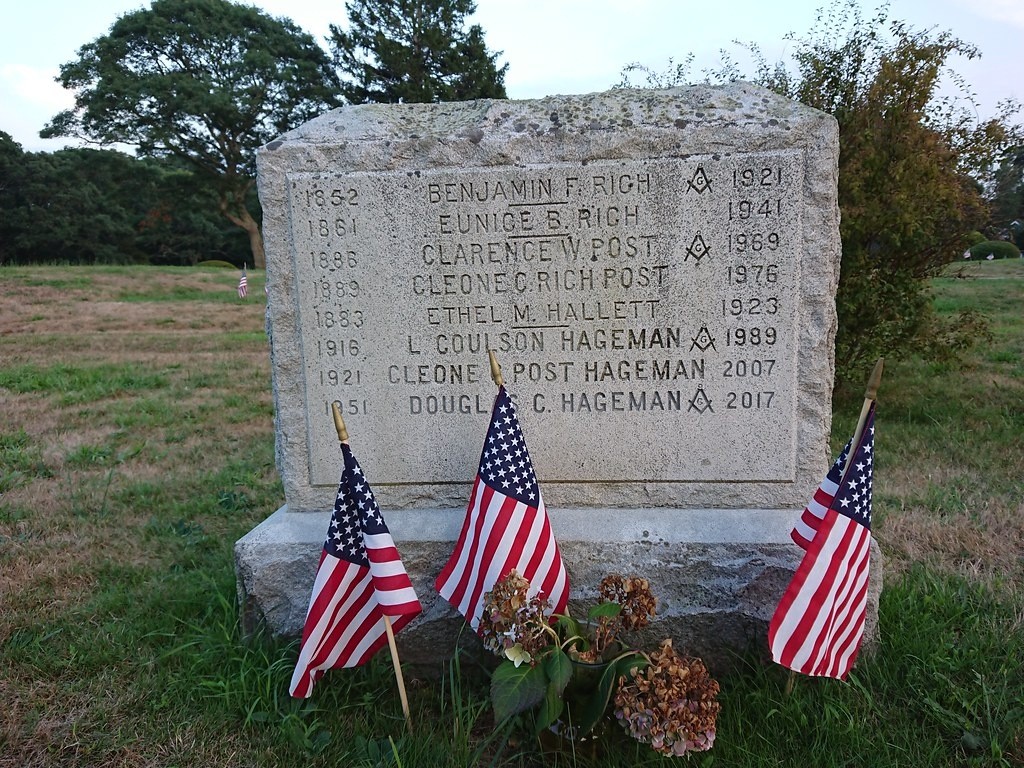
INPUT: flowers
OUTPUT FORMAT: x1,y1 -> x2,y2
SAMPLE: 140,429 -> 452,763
472,567 -> 722,757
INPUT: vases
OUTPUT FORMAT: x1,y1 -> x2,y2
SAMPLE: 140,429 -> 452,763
549,621 -> 623,721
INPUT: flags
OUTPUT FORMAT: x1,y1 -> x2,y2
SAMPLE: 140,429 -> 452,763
987,254 -> 994,260
238,269 -> 248,298
288,443 -> 423,698
964,248 -> 971,259
768,399 -> 875,680
434,384 -> 570,639
